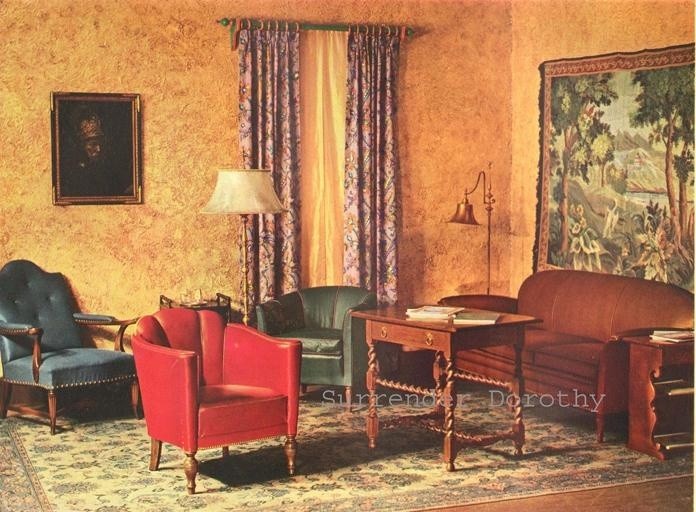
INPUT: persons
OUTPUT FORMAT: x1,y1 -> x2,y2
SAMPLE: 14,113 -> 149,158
59,113 -> 133,196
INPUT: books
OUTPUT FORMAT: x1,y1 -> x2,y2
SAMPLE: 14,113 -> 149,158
407,308 -> 456,319
453,312 -> 501,324
405,305 -> 465,316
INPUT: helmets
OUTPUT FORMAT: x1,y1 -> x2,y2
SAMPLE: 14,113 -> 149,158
79,119 -> 105,139
